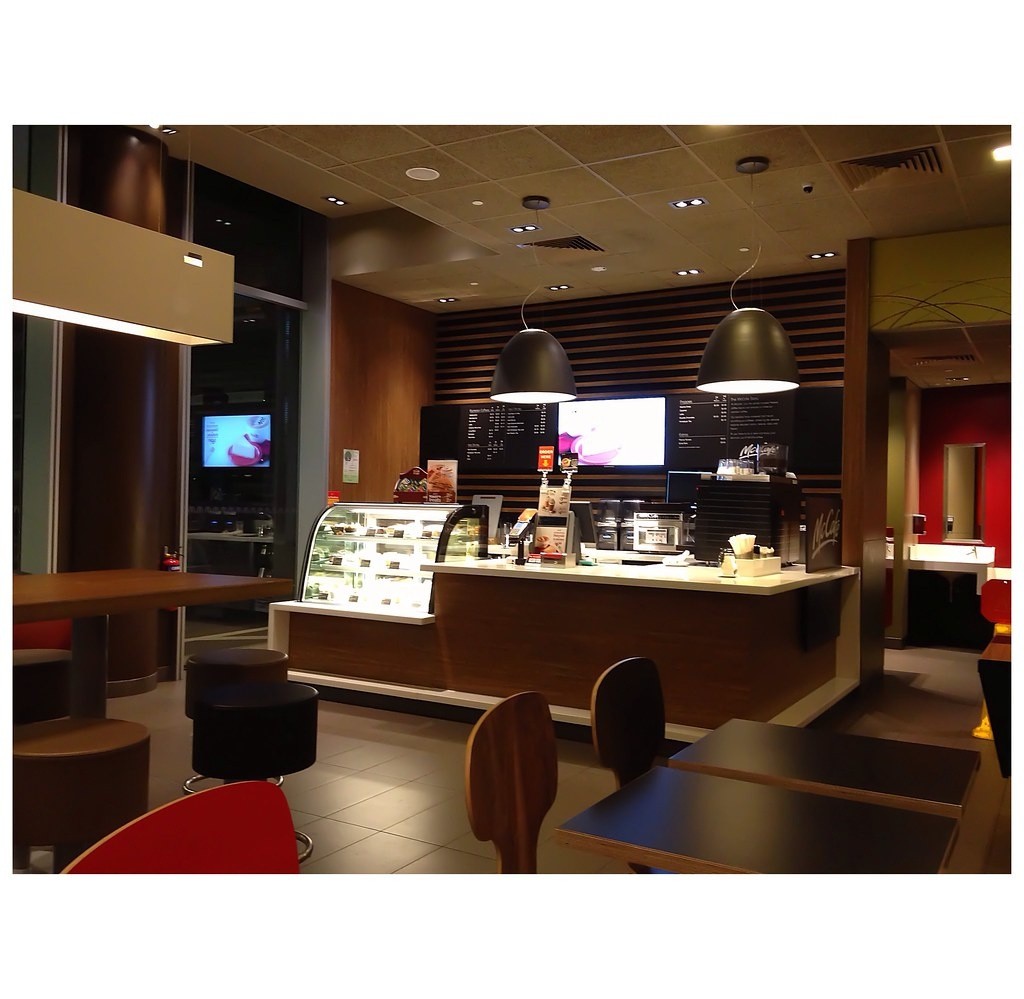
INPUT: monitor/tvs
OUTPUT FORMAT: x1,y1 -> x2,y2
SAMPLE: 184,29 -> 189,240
199,413 -> 273,470
555,394 -> 669,469
568,501 -> 597,543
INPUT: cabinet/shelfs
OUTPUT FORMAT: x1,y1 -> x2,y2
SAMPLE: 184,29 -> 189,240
308,531 -> 477,613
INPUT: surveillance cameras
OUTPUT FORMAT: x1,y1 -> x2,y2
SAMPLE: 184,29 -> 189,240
803,185 -> 813,193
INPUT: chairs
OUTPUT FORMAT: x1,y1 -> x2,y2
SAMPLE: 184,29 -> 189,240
46,782 -> 298,874
464,690 -> 560,874
592,655 -> 668,874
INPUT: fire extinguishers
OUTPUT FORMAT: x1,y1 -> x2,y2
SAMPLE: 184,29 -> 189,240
164,546 -> 185,611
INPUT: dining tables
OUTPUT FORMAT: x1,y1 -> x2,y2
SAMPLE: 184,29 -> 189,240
558,717 -> 982,873
13,566 -> 294,719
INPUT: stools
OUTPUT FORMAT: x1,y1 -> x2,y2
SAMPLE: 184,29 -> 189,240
13,643 -> 319,865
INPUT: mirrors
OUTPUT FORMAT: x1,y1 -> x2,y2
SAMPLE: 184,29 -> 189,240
943,442 -> 986,544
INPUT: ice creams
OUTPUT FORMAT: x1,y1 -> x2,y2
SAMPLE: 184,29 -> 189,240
545,496 -> 555,512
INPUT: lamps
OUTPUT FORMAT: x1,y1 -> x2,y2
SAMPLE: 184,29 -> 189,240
490,195 -> 577,405
696,157 -> 801,395
